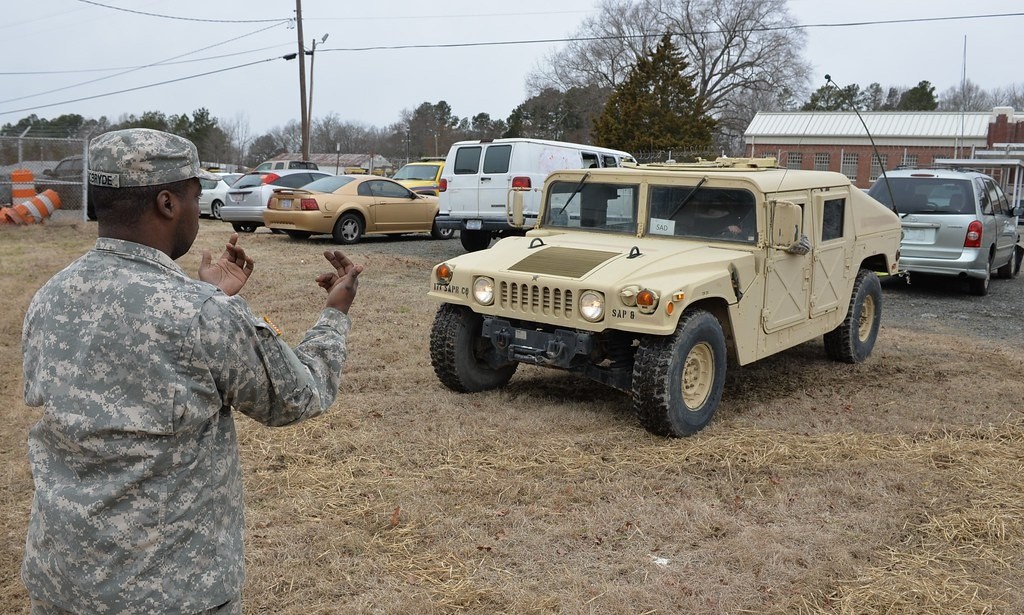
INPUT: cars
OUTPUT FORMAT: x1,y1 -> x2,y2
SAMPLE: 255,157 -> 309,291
264,174 -> 456,245
218,170 -> 339,234
199,173 -> 246,220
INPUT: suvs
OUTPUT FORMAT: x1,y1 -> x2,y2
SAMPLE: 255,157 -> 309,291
34,154 -> 84,210
434,137 -> 643,254
427,154 -> 904,440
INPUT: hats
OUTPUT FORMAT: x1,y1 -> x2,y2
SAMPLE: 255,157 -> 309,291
88,128 -> 223,189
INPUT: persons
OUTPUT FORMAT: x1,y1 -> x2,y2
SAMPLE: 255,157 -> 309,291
694,194 -> 747,244
20,128 -> 364,615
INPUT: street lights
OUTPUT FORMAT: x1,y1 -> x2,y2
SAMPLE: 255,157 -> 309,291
306,33 -> 331,151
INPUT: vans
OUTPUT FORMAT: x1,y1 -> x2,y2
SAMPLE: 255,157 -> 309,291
250,160 -> 319,173
378,156 -> 446,198
867,170 -> 1023,296
